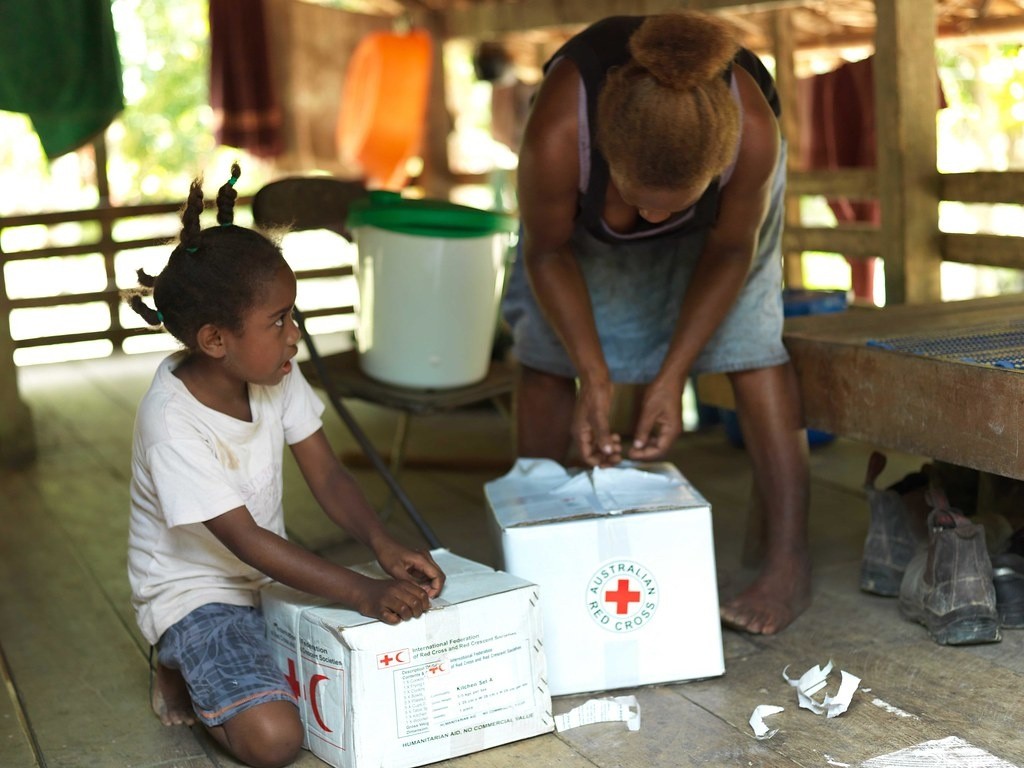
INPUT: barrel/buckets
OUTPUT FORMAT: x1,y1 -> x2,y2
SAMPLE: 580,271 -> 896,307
343,189 -> 519,391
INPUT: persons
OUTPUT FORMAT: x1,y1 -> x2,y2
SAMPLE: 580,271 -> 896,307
496,14 -> 813,637
127,164 -> 445,768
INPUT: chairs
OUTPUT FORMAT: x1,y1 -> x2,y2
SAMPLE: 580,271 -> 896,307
248,177 -> 520,550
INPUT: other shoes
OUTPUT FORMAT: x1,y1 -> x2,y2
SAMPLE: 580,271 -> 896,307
993,567 -> 1024,628
900,508 -> 1002,648
860,488 -> 929,597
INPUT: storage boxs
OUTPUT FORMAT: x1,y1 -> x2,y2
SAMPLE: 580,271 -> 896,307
726,290 -> 845,443
259,547 -> 558,768
485,462 -> 724,697
